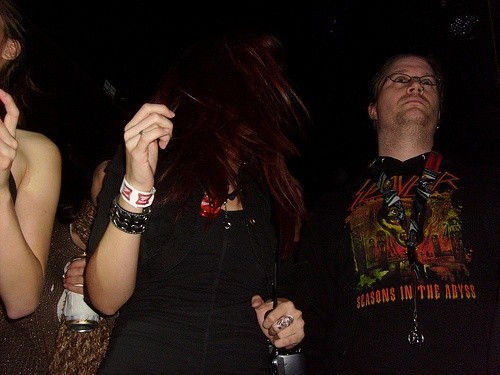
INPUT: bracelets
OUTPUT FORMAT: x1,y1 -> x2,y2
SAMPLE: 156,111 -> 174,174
119,175 -> 156,209
109,197 -> 154,234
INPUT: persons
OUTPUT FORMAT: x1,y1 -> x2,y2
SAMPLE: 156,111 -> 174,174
0,0 -> 61,375
39,154 -> 121,375
299,54 -> 500,375
84,31 -> 306,375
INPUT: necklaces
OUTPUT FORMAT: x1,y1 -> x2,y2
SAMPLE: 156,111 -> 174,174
223,190 -> 237,229
375,150 -> 443,345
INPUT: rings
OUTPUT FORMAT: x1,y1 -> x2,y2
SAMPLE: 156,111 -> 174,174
277,315 -> 293,330
140,130 -> 144,135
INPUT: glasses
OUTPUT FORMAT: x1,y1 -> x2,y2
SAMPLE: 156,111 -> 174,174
374,73 -> 441,102
